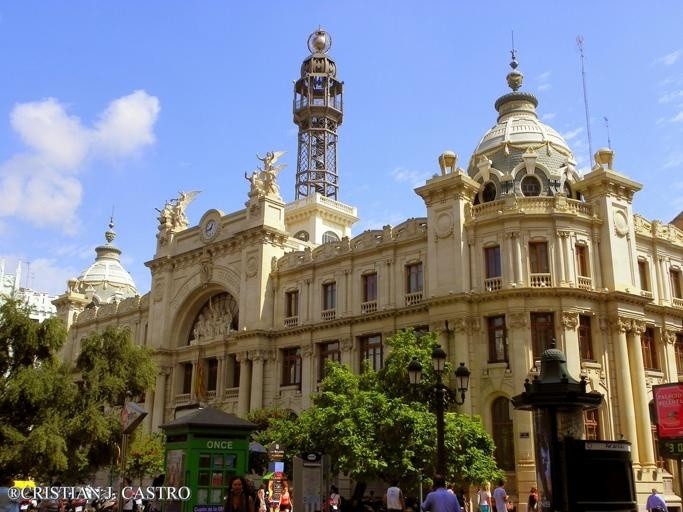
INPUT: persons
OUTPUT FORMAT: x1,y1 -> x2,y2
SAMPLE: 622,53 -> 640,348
222,476 -> 295,512
190,294 -> 232,343
120,476 -> 134,511
157,198 -> 174,219
492,478 -> 508,512
645,488 -> 668,511
256,149 -> 274,171
526,486 -> 538,511
476,482 -> 492,512
322,478 -> 418,511
446,484 -> 455,497
165,197 -> 183,228
170,189 -> 187,218
242,170 -> 266,198
256,163 -> 276,197
420,473 -> 462,512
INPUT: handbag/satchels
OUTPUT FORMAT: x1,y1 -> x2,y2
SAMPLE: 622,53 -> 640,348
477,494 -> 481,504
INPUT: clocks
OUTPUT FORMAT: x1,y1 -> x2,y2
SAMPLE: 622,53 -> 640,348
204,219 -> 216,238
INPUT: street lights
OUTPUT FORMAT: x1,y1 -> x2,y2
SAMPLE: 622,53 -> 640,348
406,343 -> 470,473
